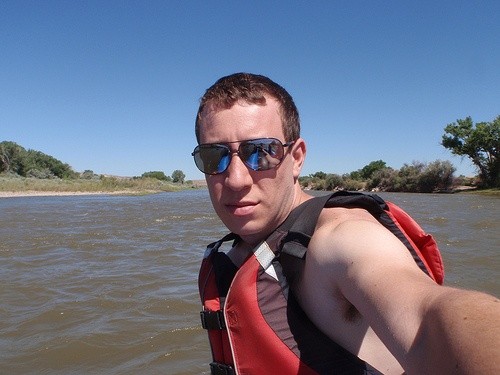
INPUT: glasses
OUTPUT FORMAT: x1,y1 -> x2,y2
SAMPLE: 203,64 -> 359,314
191,138 -> 295,176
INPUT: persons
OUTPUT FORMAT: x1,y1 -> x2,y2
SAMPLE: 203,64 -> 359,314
191,73 -> 500,375
